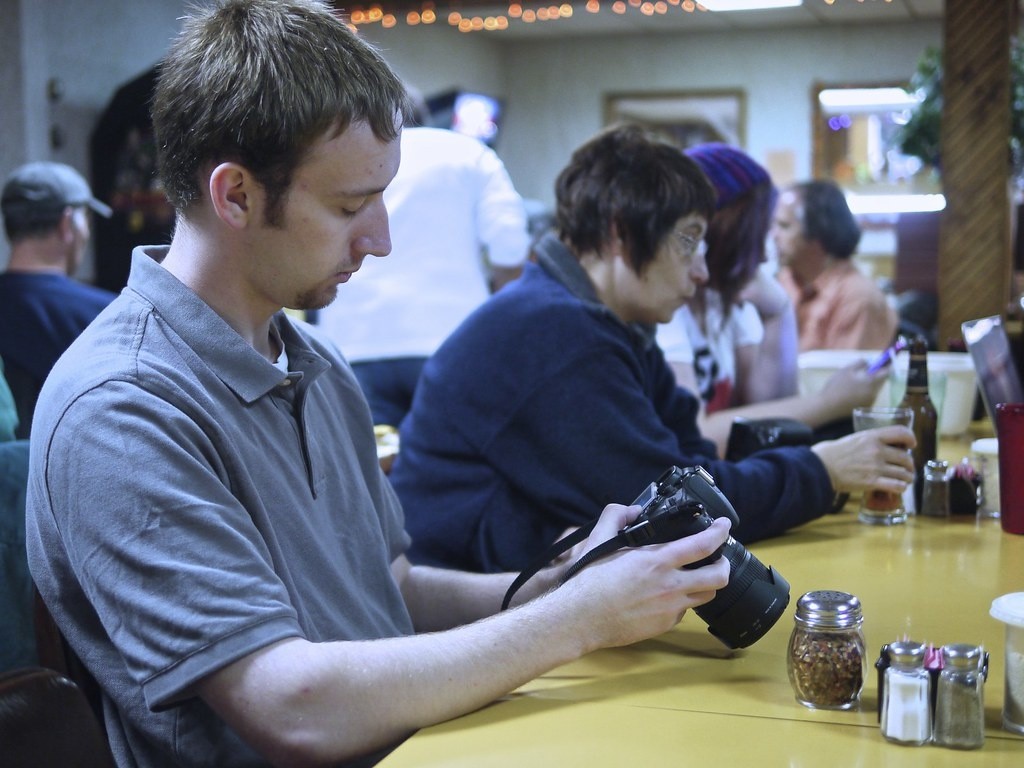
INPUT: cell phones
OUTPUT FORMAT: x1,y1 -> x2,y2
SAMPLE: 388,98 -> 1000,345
869,336 -> 907,375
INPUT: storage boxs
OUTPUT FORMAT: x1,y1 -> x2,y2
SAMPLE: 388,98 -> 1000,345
796,345 -> 980,436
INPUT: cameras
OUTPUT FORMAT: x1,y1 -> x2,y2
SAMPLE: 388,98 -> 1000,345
625,465 -> 791,649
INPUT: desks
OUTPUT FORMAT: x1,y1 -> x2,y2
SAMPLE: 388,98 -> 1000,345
364,410 -> 1024,768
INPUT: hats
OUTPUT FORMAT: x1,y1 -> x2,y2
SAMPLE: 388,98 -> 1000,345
1,161 -> 114,221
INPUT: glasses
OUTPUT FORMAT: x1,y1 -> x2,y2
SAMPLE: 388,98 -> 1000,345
672,228 -> 709,260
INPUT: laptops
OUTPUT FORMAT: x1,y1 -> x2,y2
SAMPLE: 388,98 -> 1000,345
962,315 -> 1024,436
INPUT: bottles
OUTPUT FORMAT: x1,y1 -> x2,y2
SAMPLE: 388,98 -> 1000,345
935,643 -> 984,749
972,454 -> 1000,518
949,464 -> 979,516
787,590 -> 868,710
880,641 -> 932,746
895,340 -> 937,515
923,460 -> 950,516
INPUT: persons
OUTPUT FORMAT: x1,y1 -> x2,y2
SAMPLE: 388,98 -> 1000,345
0,164 -> 119,439
24,1 -> 730,768
387,123 -> 919,577
649,142 -> 892,456
769,179 -> 898,354
309,86 -> 530,432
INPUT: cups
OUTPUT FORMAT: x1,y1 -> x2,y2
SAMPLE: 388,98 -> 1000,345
1002,623 -> 1024,735
995,403 -> 1024,535
853,407 -> 914,525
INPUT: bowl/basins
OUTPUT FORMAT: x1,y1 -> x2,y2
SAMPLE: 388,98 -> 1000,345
797,350 -> 976,438
